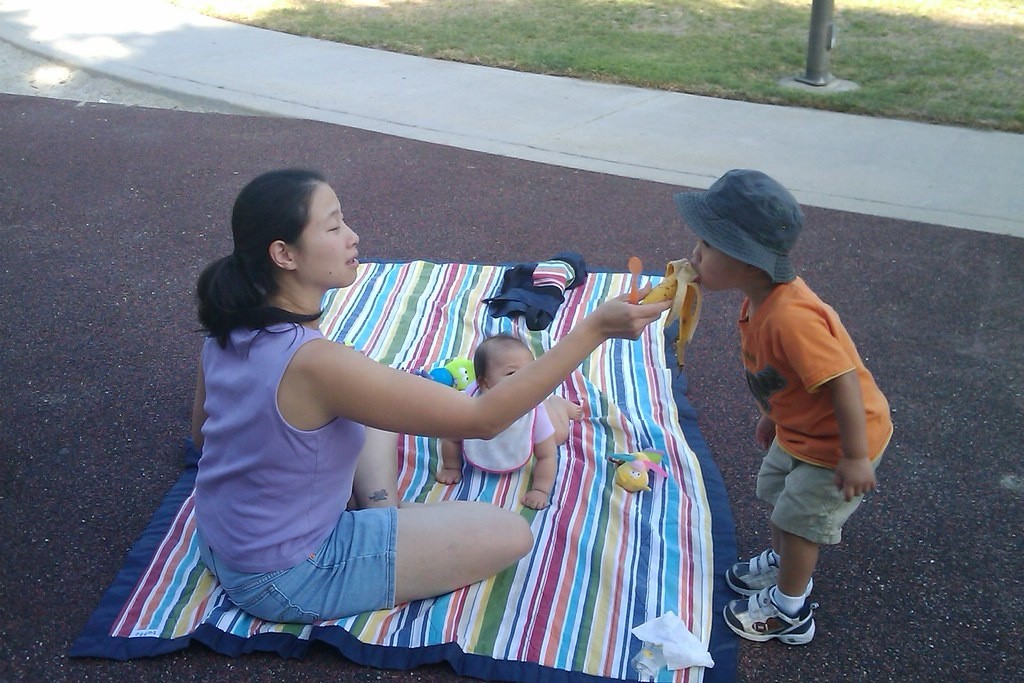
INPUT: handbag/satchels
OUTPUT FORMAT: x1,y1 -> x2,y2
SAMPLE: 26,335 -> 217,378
482,260 -> 576,330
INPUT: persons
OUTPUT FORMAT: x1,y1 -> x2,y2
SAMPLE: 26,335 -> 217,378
672,169 -> 894,645
191,168 -> 673,625
435,332 -> 582,510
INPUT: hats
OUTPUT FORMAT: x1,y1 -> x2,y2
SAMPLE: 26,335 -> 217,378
548,252 -> 587,288
672,168 -> 806,284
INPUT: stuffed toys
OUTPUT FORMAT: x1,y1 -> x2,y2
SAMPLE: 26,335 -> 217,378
608,448 -> 668,492
422,358 -> 476,390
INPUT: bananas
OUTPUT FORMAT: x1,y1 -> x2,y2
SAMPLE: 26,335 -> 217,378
641,257 -> 701,366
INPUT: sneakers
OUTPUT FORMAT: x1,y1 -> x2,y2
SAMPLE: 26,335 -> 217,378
727,549 -> 814,600
721,586 -> 819,646
